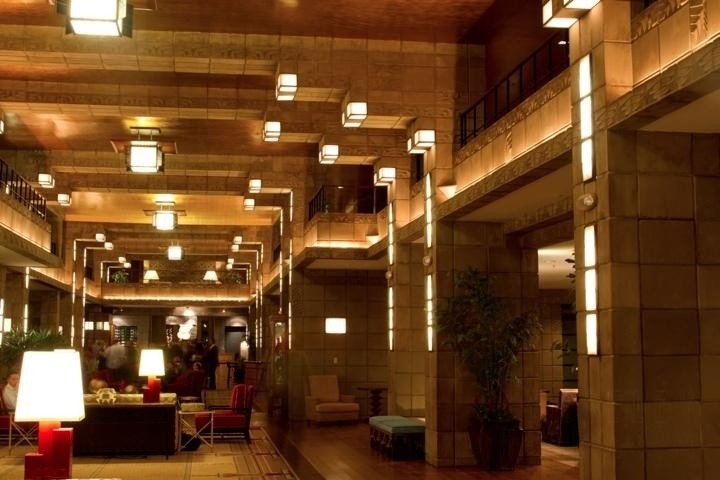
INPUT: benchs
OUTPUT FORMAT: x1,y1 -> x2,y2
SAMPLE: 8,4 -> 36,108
368,415 -> 426,459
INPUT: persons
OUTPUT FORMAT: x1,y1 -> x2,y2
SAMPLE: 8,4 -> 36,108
88,336 -> 219,394
2,373 -> 19,415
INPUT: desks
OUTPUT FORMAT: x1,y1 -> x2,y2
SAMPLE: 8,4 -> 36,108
8,411 -> 39,457
356,386 -> 389,415
177,409 -> 217,452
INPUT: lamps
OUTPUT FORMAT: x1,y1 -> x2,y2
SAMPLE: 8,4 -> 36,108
144,269 -> 159,279
167,246 -> 185,261
95,233 -> 106,243
243,199 -> 256,210
275,86 -> 296,101
319,151 -> 334,164
13,347 -> 87,480
265,121 -> 280,136
322,145 -> 340,158
104,242 -> 113,250
49,1 -> 155,38
225,257 -> 235,269
379,167 -> 396,181
203,266 -> 218,280
262,131 -> 280,141
416,130 -> 436,147
118,257 -> 127,264
341,112 -> 363,127
231,235 -> 243,252
125,140 -> 166,173
124,263 -> 131,268
346,102 -> 367,119
373,174 -> 390,186
277,74 -> 298,91
406,137 -> 428,153
57,193 -> 72,208
153,211 -> 177,230
249,180 -> 261,193
137,348 -> 166,403
37,173 -> 55,189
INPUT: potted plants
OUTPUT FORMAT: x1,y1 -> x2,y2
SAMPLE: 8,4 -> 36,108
432,261 -> 543,471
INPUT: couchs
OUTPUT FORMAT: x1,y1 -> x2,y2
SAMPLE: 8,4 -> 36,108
60,402 -> 176,461
545,389 -> 578,446
0,383 -> 39,435
302,374 -> 361,427
195,383 -> 255,441
